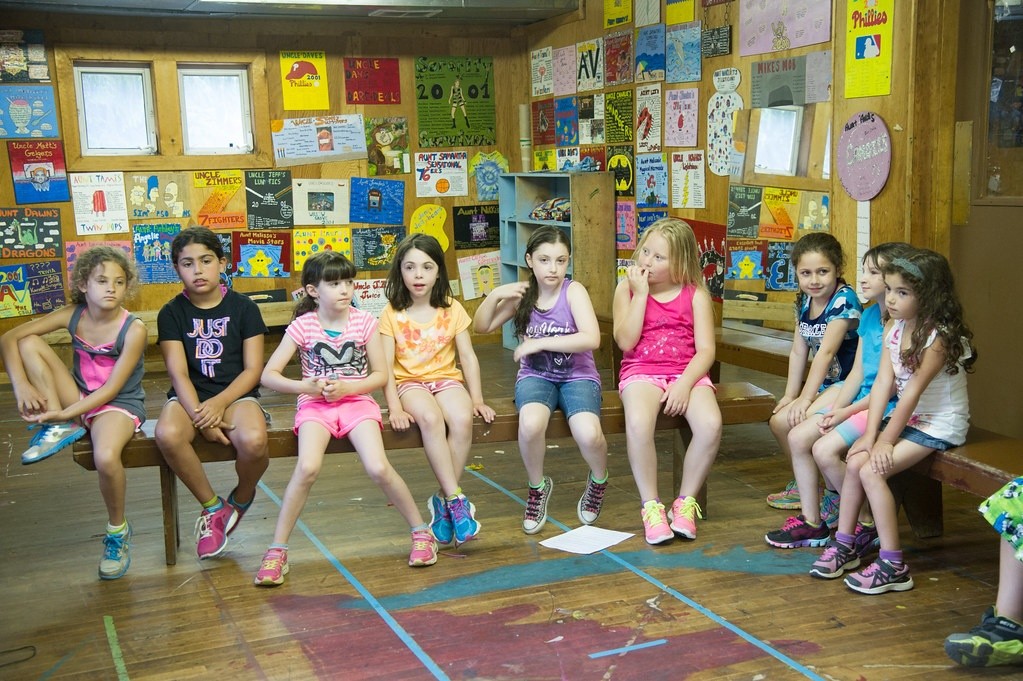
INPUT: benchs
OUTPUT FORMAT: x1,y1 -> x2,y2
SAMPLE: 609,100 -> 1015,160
855,425 -> 1023,539
21,302 -> 302,372
595,311 -> 818,385
74,380 -> 777,566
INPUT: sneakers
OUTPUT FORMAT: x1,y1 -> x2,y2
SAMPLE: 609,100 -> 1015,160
521,476 -> 554,535
427,491 -> 454,545
855,521 -> 881,559
408,528 -> 439,567
765,514 -> 832,550
445,493 -> 481,544
193,495 -> 239,560
98,519 -> 133,580
843,556 -> 914,595
809,540 -> 861,580
819,489 -> 840,529
767,479 -> 802,510
576,469 -> 609,526
225,486 -> 257,538
254,548 -> 290,587
20,418 -> 87,466
640,500 -> 675,545
666,496 -> 703,541
944,604 -> 1023,669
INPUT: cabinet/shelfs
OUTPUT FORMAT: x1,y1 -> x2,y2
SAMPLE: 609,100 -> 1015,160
497,171 -> 617,371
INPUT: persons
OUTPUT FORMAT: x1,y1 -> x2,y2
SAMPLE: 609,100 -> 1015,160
943,475 -> 1023,669
253,250 -> 438,585
613,218 -> 723,544
764,240 -> 911,557
154,228 -> 270,560
376,233 -> 497,544
0,246 -> 149,580
473,225 -> 609,535
808,248 -> 972,595
766,232 -> 864,528
